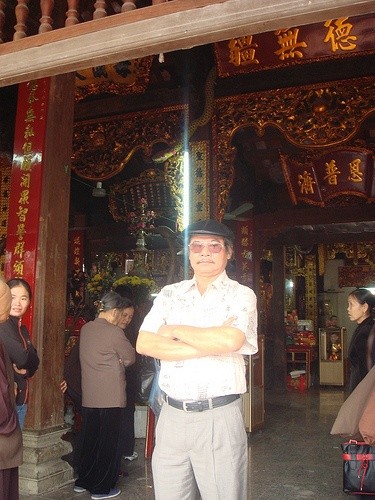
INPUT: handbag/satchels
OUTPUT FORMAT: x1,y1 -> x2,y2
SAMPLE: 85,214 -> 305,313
341,440 -> 374,497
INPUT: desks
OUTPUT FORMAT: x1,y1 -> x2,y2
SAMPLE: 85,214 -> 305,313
287,344 -> 318,389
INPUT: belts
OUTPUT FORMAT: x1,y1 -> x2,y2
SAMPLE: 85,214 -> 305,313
155,386 -> 240,414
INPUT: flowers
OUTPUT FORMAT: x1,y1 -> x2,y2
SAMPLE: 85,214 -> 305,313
111,276 -> 155,306
87,274 -> 108,309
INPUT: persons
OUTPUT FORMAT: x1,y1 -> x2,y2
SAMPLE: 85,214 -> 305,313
327,315 -> 341,330
0,281 -> 23,500
347,289 -> 375,394
6,277 -> 40,438
136,220 -> 259,500
60,291 -> 137,500
330,365 -> 375,495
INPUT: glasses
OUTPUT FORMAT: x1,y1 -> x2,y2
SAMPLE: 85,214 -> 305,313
180,219 -> 237,243
186,242 -> 227,254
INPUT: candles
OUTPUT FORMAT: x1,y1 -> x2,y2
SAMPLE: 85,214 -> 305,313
127,197 -> 157,233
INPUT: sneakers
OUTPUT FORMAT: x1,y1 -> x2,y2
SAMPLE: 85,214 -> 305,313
74,485 -> 87,492
91,489 -> 121,499
124,450 -> 140,461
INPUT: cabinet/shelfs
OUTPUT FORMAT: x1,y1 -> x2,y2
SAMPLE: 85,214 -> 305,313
318,327 -> 347,389
